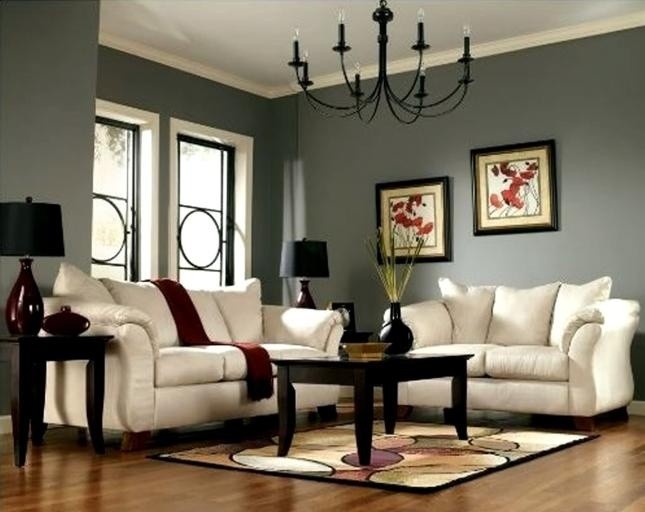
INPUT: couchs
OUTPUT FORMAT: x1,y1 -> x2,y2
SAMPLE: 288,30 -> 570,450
380,299 -> 640,431
37,280 -> 344,451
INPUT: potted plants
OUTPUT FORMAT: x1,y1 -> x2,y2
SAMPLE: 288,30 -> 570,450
364,229 -> 424,358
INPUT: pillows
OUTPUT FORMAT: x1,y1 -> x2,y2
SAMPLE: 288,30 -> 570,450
547,276 -> 612,345
438,278 -> 495,343
485,282 -> 561,346
185,288 -> 233,344
211,278 -> 264,344
97,278 -> 184,348
53,261 -> 116,305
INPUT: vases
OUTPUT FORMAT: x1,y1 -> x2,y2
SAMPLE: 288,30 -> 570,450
41,304 -> 90,336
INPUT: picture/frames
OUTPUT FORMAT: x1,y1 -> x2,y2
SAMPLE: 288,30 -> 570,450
470,140 -> 557,235
375,177 -> 451,265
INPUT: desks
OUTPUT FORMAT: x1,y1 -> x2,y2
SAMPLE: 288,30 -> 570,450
269,354 -> 475,465
340,331 -> 374,343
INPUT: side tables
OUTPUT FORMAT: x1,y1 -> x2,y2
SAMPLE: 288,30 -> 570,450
0,336 -> 114,468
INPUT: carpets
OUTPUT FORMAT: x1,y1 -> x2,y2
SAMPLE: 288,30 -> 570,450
146,419 -> 599,493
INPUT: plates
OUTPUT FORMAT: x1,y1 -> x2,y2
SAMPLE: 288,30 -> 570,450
340,341 -> 392,353
341,351 -> 391,362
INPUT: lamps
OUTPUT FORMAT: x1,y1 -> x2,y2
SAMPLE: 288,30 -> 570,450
288,1 -> 474,124
0,197 -> 64,338
279,238 -> 329,309
